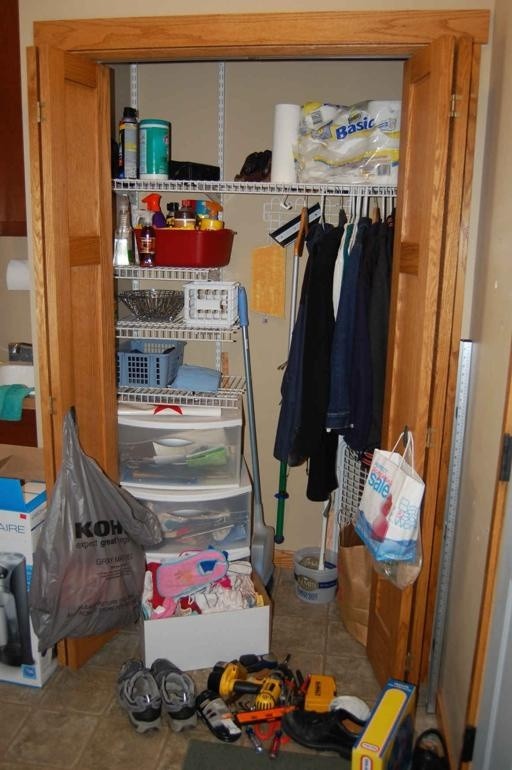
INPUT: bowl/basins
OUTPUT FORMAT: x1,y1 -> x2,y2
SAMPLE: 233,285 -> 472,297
121,290 -> 183,323
152,439 -> 193,456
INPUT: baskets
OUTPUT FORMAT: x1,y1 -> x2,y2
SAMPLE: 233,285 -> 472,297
115,339 -> 184,387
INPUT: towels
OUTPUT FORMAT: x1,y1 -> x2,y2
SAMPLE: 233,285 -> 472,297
0,382 -> 35,423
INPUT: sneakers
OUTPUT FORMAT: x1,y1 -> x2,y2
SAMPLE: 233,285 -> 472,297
150,658 -> 197,732
116,658 -> 162,733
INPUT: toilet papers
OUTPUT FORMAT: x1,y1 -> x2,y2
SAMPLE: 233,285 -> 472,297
6,258 -> 32,293
300,100 -> 399,183
270,102 -> 299,183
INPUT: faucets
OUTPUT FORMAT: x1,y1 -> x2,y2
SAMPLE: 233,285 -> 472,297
8,341 -> 32,358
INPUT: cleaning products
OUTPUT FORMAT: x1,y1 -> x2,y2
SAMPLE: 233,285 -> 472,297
141,193 -> 169,227
199,201 -> 225,231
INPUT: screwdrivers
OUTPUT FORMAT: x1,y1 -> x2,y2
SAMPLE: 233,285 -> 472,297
269,687 -> 291,760
233,702 -> 263,756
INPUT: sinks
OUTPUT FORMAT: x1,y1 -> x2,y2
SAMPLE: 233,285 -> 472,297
0,365 -> 35,394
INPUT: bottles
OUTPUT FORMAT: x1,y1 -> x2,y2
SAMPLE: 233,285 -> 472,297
139,212 -> 158,267
180,198 -> 191,212
113,196 -> 134,268
119,107 -> 138,179
166,202 -> 179,226
173,200 -> 193,226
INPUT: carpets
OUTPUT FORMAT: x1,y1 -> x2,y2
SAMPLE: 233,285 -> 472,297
183,739 -> 351,770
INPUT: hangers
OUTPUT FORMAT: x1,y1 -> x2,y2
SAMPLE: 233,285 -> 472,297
294,184 -> 396,229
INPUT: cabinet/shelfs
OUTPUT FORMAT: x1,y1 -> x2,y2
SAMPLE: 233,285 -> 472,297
111,178 -> 397,410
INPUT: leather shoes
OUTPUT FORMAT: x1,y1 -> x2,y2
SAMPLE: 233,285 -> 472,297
281,709 -> 367,760
411,728 -> 449,770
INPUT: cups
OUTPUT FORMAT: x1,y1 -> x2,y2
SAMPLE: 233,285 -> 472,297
139,119 -> 170,178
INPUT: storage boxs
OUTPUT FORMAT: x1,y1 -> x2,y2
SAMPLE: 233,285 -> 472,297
0,477 -> 46,591
117,455 -> 252,565
141,581 -> 272,670
0,395 -> 38,448
132,228 -> 238,267
117,392 -> 244,488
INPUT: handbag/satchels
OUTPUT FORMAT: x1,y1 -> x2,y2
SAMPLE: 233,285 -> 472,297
354,431 -> 425,562
29,413 -> 163,652
